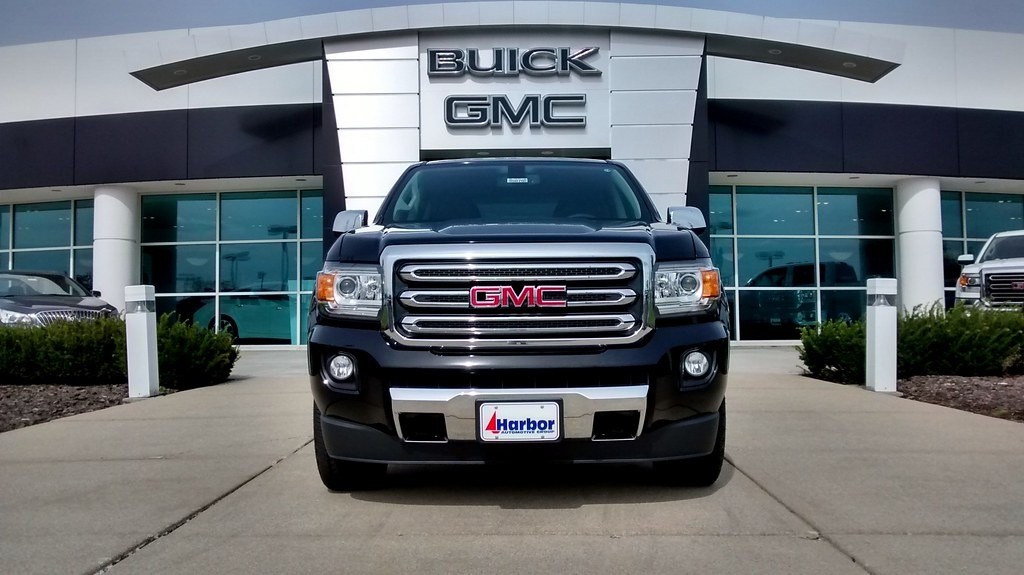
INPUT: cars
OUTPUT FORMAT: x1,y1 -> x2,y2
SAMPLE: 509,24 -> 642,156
0,271 -> 120,326
192,282 -> 309,343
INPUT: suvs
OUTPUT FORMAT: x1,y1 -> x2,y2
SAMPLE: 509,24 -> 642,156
728,261 -> 862,326
305,156 -> 733,492
954,230 -> 1024,312
